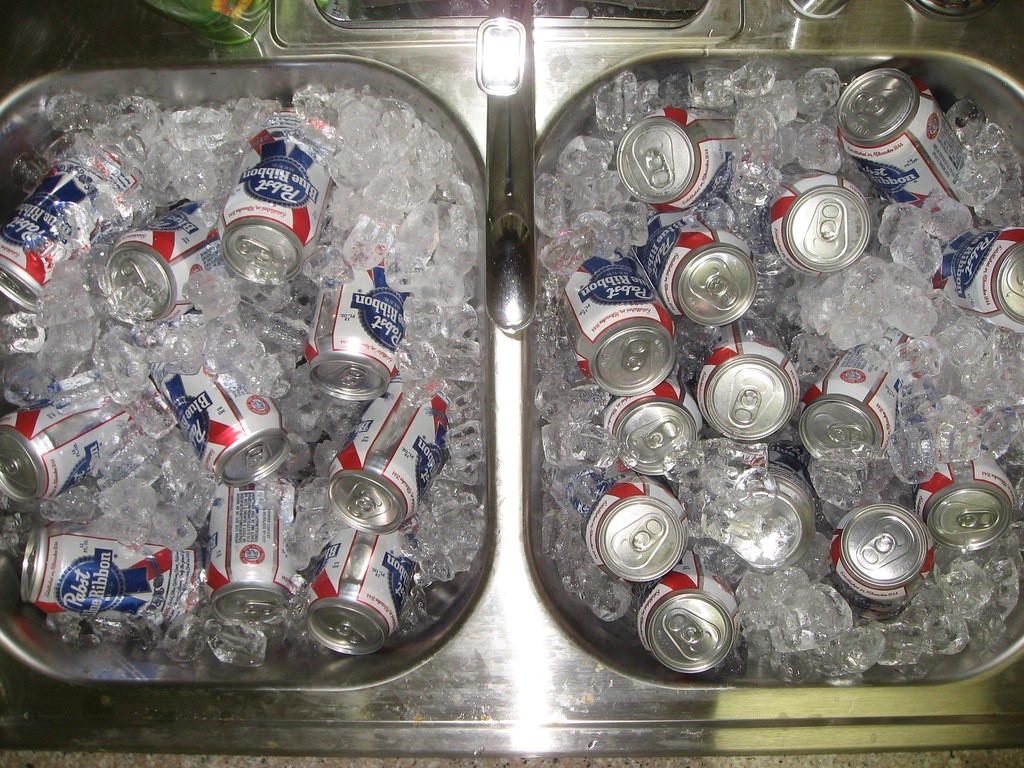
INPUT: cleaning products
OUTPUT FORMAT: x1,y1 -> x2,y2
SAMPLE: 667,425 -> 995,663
140,0 -> 273,46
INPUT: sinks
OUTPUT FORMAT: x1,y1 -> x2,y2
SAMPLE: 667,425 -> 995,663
313,1 -> 713,29
0,51 -> 508,693
508,46 -> 1024,693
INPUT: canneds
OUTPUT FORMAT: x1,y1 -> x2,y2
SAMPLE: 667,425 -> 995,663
560,68 -> 1024,676
0,108 -> 449,654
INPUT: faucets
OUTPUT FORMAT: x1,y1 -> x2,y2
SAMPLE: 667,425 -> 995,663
475,0 -> 540,337
